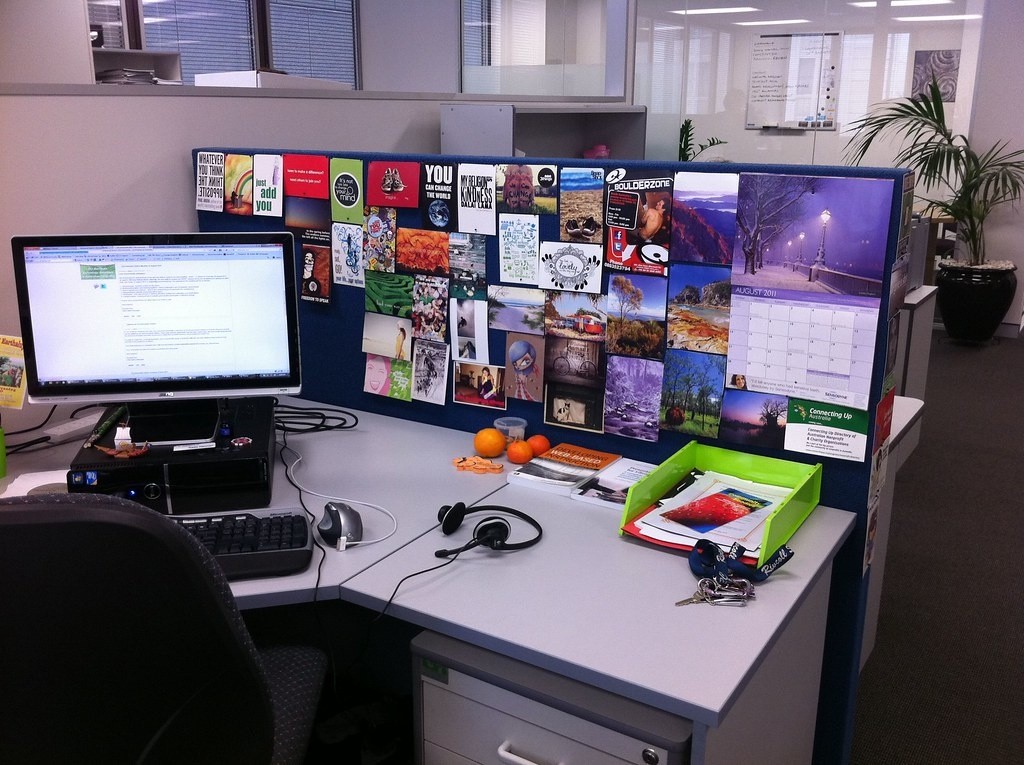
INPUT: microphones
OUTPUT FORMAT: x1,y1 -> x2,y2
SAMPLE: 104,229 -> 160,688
435,531 -> 494,558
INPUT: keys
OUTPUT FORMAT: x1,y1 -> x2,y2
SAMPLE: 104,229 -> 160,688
674,591 -> 718,607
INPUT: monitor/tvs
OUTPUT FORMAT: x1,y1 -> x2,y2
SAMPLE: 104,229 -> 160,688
10,231 -> 302,445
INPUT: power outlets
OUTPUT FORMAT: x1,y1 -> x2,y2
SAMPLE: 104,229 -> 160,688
40,406 -> 105,447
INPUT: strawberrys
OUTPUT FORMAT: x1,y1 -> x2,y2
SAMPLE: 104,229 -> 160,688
660,492 -> 750,526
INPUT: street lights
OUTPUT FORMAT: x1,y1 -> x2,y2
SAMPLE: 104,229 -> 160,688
815,208 -> 831,266
798,231 -> 805,262
788,239 -> 792,262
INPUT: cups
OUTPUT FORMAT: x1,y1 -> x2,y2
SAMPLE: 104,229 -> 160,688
494,417 -> 527,451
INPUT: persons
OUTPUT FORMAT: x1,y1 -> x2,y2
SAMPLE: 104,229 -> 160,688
410,276 -> 445,342
1,367 -> 16,386
420,348 -> 438,379
728,374 -> 748,391
393,319 -> 406,360
638,197 -> 668,244
478,367 -> 497,399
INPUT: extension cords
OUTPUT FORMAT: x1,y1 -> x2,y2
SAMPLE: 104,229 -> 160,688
44,412 -> 104,445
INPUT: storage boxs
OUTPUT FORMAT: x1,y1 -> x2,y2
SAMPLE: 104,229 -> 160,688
619,439 -> 823,569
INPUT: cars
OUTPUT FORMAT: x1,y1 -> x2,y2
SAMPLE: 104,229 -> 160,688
448,233 -> 473,252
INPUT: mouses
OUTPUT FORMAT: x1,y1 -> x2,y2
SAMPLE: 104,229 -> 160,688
317,502 -> 363,551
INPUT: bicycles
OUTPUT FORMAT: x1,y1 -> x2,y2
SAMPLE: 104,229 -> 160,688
553,346 -> 597,380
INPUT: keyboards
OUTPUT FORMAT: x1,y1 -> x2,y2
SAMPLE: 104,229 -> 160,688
171,507 -> 312,582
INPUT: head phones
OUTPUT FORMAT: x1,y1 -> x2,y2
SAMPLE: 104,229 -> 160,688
437,502 -> 543,551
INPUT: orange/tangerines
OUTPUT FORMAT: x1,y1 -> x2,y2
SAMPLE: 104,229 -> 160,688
527,434 -> 550,458
507,439 -> 533,464
474,427 -> 506,457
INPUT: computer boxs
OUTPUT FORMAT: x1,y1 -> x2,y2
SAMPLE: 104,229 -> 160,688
67,395 -> 277,516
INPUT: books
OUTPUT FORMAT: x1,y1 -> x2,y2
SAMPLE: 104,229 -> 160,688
507,442 -> 622,497
570,457 -> 659,511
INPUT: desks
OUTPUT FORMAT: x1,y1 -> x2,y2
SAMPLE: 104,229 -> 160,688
0,389 -> 859,765
911,200 -> 955,261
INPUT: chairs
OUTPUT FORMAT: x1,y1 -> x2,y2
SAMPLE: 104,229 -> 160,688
0,493 -> 275,765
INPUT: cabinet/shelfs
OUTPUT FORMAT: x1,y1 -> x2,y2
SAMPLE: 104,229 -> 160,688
415,634 -> 694,765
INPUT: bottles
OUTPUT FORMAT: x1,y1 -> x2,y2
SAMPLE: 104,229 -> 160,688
0,413 -> 6,478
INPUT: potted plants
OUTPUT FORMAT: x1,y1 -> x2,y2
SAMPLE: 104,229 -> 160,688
840,83 -> 1024,349
